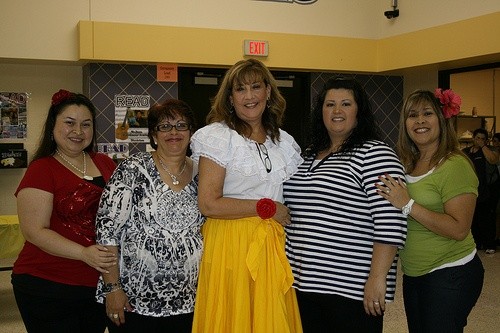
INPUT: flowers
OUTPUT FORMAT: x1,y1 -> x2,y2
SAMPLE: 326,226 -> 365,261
434,89 -> 462,119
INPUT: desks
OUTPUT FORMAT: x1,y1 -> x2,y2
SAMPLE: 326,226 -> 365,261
0,215 -> 26,267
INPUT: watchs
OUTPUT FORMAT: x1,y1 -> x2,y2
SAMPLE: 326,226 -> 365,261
402,199 -> 415,215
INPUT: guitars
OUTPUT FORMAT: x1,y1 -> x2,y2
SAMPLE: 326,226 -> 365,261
115,107 -> 130,140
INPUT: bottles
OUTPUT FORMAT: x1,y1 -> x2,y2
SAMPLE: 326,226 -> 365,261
472,106 -> 478,118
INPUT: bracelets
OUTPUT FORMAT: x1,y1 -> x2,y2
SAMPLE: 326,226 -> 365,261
102,278 -> 121,294
481,145 -> 485,148
256,198 -> 276,219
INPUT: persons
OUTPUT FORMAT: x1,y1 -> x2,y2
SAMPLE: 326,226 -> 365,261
461,128 -> 500,253
94,99 -> 206,333
129,110 -> 149,128
376,90 -> 484,333
189,59 -> 304,333
282,78 -> 408,333
10,88 -> 117,333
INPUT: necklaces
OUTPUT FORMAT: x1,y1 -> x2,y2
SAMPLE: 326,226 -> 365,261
156,153 -> 186,185
56,149 -> 93,181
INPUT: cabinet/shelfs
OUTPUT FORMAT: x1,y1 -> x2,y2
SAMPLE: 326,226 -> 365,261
454,114 -> 497,144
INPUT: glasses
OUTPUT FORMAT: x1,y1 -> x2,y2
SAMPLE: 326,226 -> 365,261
255,142 -> 273,173
152,122 -> 192,132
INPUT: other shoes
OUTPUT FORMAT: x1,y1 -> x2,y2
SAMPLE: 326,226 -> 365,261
486,249 -> 496,254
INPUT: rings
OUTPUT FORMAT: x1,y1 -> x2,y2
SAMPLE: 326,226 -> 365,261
374,302 -> 379,304
386,188 -> 390,195
113,314 -> 118,318
